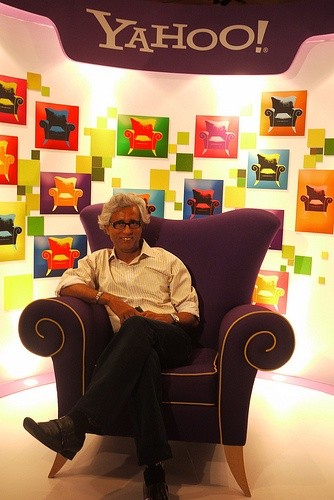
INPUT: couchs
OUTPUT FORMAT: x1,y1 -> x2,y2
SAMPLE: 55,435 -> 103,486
17,203 -> 298,497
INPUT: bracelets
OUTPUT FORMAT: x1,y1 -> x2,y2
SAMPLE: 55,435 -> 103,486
95,291 -> 103,302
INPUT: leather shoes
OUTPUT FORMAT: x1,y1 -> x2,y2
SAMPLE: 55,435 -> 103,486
22,415 -> 85,460
142,462 -> 170,500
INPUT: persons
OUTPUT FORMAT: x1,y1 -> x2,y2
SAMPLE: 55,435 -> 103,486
22,192 -> 200,500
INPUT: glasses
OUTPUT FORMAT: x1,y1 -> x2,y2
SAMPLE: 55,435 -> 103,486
106,220 -> 143,230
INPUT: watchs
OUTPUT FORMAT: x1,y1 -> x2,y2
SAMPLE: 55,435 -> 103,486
170,313 -> 180,323
